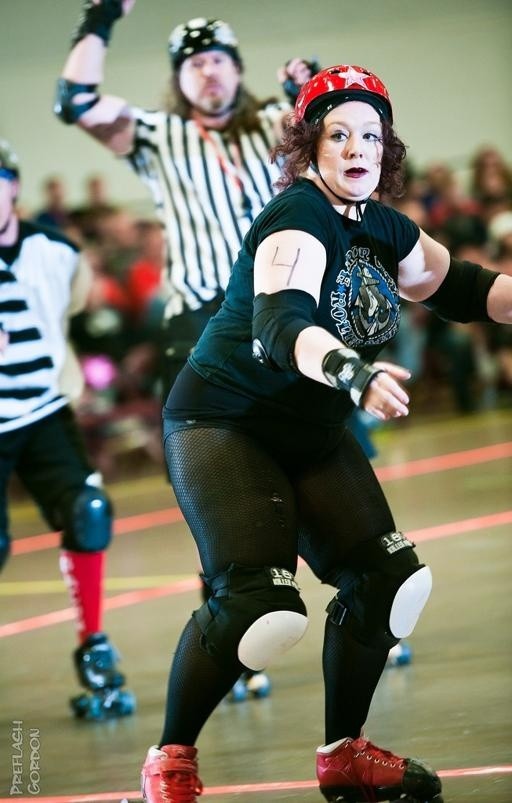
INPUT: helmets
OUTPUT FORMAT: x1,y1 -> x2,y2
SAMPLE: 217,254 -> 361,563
168,17 -> 236,67
295,65 -> 393,127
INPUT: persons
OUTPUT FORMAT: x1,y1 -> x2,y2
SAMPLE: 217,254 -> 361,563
1,1 -> 510,513
48,1 -> 414,709
139,60 -> 509,803
1,142 -> 129,692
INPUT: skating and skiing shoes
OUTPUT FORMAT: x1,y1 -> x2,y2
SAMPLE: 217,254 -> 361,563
71,633 -> 135,719
316,731 -> 443,803
142,745 -> 202,803
